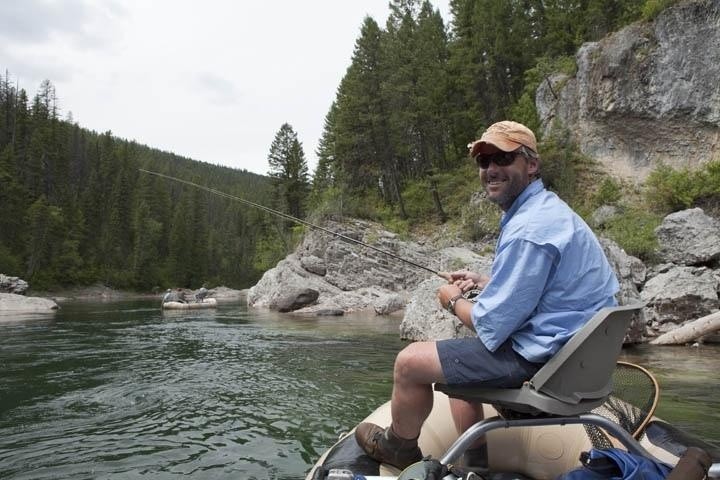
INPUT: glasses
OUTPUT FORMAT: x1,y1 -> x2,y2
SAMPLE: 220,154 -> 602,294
475,149 -> 529,167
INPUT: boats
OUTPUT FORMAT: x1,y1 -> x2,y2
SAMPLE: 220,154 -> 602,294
163,297 -> 217,309
305,382 -> 720,480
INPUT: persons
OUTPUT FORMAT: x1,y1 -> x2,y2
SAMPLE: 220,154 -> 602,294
193,286 -> 208,303
163,288 -> 174,301
354,120 -> 622,480
174,287 -> 188,303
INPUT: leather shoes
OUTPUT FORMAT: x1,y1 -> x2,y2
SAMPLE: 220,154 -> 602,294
354,421 -> 424,470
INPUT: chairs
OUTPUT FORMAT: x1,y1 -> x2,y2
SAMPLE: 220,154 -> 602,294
432,297 -> 656,469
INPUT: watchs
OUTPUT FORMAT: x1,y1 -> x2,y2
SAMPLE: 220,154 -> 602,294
446,294 -> 463,316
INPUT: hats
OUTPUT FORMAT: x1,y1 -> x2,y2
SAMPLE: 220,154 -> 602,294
470,120 -> 540,159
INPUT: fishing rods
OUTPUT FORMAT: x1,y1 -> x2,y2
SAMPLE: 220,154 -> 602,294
138,169 -> 482,299
